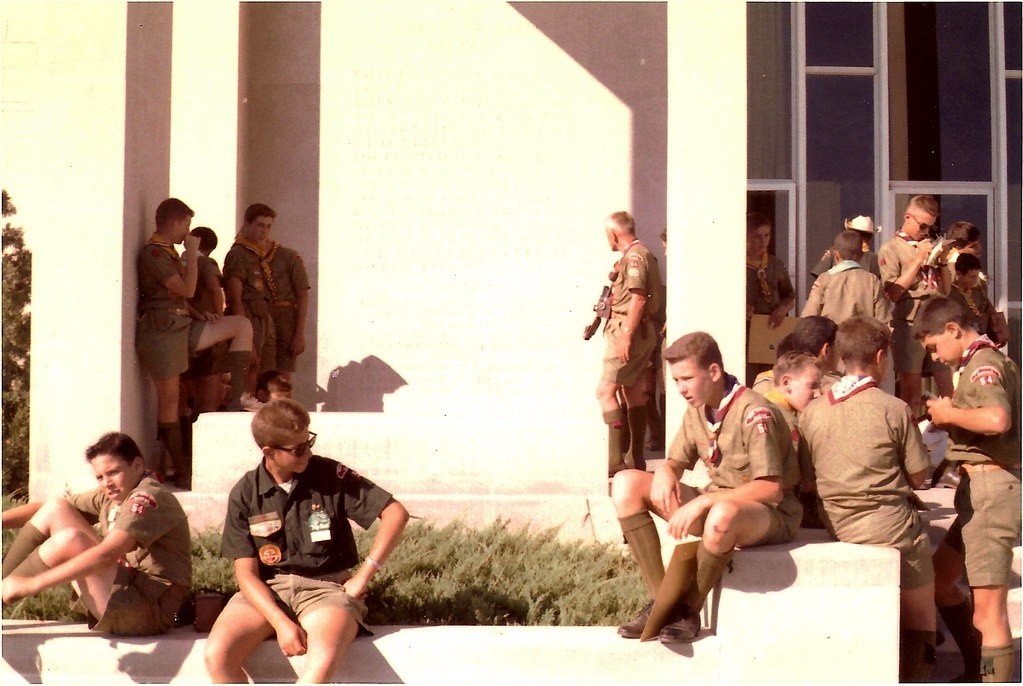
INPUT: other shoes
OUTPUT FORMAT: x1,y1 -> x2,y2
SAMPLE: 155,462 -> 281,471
609,462 -> 627,478
949,675 -> 982,683
624,459 -> 646,471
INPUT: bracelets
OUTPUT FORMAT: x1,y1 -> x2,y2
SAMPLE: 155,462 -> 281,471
365,556 -> 383,570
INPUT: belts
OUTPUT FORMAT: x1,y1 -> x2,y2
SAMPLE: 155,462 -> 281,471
610,311 -> 648,338
271,300 -> 293,308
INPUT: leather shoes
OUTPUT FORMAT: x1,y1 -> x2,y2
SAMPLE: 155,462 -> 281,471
931,468 -> 961,489
223,392 -> 266,411
660,605 -> 700,643
617,599 -> 659,639
174,471 -> 190,488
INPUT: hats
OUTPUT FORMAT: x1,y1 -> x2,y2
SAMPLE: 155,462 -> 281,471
845,215 -> 881,235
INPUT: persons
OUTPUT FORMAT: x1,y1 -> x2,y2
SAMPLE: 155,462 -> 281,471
949,253 -> 1007,349
769,351 -> 826,531
800,233 -> 895,399
618,227 -> 666,459
792,316 -> 844,395
913,296 -> 1021,682
222,202 -> 310,412
597,211 -> 662,497
877,195 -> 962,488
798,316 -> 937,683
203,398 -> 410,684
613,331 -> 802,644
751,333 -> 796,394
2,432 -> 193,638
946,221 -> 987,294
180,227 -> 230,479
135,198 -> 253,490
810,215 -> 881,280
746,213 -> 795,390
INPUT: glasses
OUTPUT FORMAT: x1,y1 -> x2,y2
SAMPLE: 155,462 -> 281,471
268,431 -> 317,457
905,213 -> 936,230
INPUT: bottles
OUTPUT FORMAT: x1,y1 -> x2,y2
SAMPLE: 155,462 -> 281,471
921,425 -> 947,468
